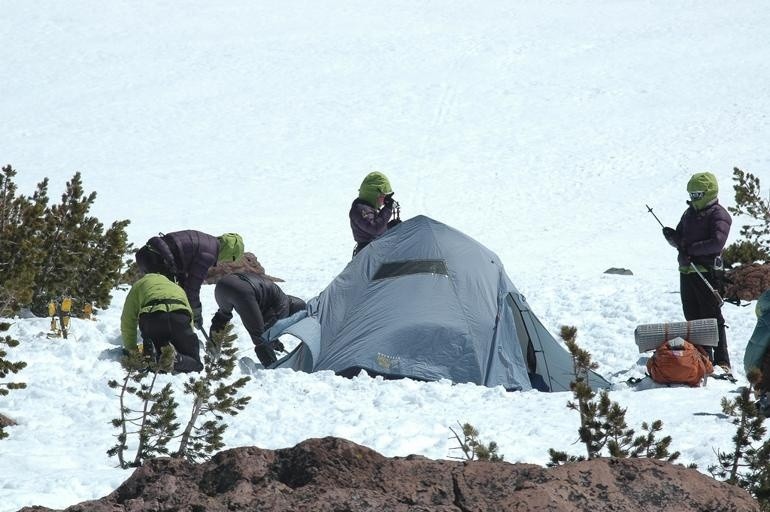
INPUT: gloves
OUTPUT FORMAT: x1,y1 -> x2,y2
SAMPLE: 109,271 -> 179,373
275,340 -> 285,352
384,192 -> 394,210
194,318 -> 203,330
678,254 -> 690,266
387,219 -> 401,230
663,227 -> 676,241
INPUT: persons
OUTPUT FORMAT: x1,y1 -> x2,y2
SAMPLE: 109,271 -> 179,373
136,229 -> 242,330
349,170 -> 401,261
661,172 -> 732,380
206,274 -> 306,368
120,273 -> 203,374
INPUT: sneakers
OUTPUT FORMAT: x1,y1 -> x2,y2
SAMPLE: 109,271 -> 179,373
143,339 -> 156,362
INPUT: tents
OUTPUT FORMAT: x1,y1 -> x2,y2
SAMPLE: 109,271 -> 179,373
262,216 -> 613,397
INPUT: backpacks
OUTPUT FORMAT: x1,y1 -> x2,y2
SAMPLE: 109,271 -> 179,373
645,320 -> 715,387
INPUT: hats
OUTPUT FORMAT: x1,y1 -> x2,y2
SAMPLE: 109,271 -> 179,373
359,172 -> 392,208
687,172 -> 718,210
216,233 -> 245,265
